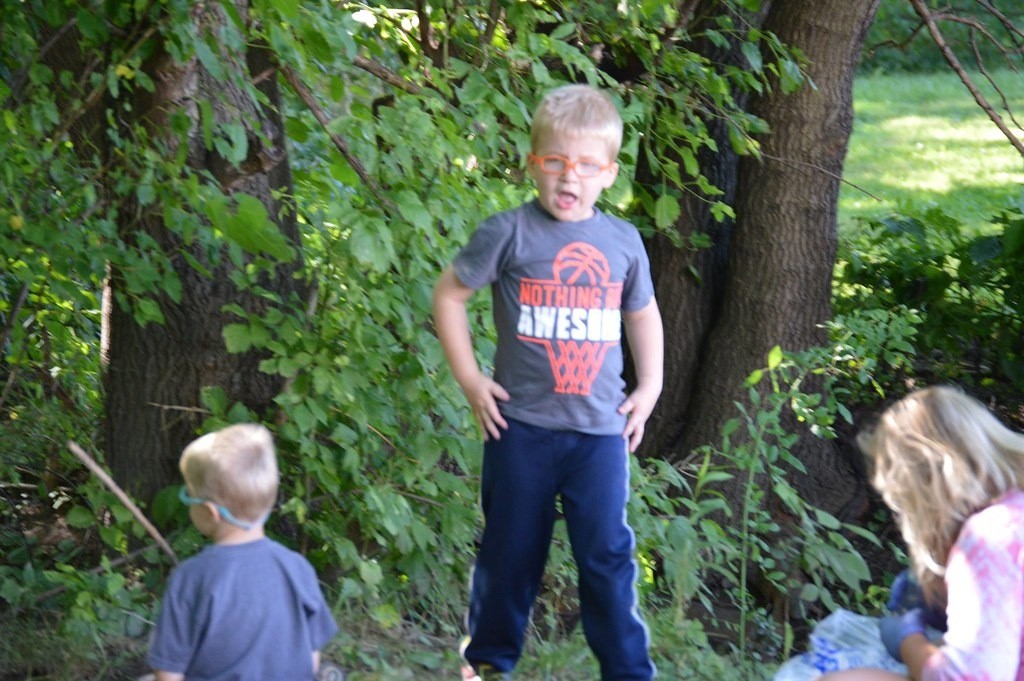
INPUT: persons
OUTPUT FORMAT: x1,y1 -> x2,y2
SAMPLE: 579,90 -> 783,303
145,426 -> 338,681
433,85 -> 665,681
774,386 -> 1024,681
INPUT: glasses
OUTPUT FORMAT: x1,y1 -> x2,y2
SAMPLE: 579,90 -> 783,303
527,153 -> 614,179
178,485 -> 263,531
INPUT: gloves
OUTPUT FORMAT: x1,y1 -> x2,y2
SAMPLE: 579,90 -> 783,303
885,570 -> 929,613
879,607 -> 928,663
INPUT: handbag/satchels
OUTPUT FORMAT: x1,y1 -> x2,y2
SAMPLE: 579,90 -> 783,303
773,606 -> 944,681
